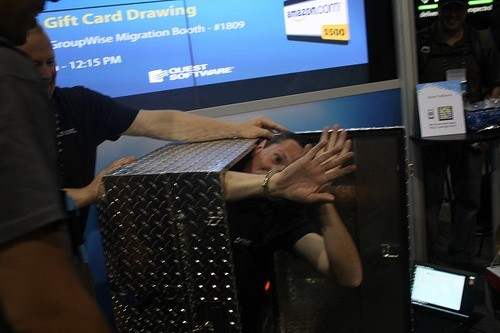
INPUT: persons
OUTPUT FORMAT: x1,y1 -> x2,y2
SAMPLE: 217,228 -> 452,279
21,18 -> 291,333
222,124 -> 363,333
0,0 -> 111,333
415,0 -> 500,272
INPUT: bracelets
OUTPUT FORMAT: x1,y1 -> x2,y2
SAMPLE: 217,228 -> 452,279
263,168 -> 282,202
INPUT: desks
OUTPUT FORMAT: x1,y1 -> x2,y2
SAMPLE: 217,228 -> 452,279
408,128 -> 500,264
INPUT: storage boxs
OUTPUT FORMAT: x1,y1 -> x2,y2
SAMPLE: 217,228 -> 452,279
463,106 -> 500,133
95,125 -> 415,333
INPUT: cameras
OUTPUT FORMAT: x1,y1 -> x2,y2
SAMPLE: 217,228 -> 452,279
409,262 -> 481,314
